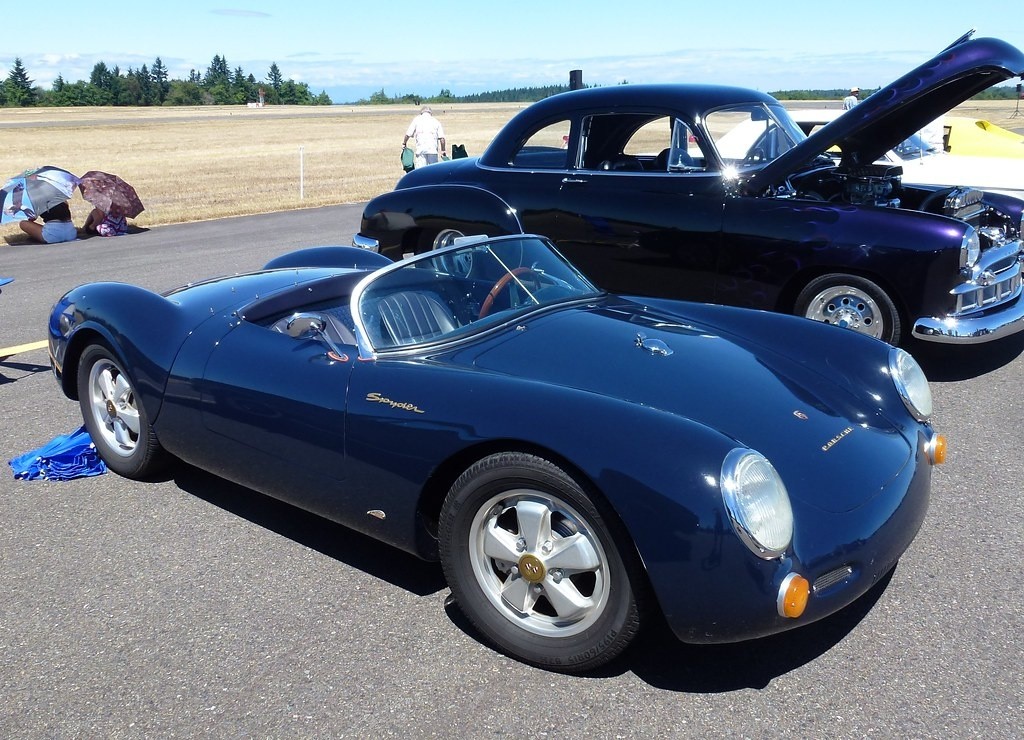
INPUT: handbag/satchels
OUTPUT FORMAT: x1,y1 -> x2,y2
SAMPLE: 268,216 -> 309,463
401,148 -> 415,173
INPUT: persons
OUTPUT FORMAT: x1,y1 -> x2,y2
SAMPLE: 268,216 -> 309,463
842,87 -> 859,110
79,207 -> 129,238
19,200 -> 78,244
401,105 -> 447,170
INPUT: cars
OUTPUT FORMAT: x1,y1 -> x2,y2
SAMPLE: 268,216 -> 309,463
353,29 -> 1024,347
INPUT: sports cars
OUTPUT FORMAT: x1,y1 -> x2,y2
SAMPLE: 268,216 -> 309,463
47,233 -> 947,675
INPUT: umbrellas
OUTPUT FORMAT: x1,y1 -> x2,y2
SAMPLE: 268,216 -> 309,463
0,164 -> 84,226
78,171 -> 146,220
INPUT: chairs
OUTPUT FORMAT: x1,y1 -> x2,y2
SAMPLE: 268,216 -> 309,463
377,292 -> 458,346
270,312 -> 358,345
597,153 -> 644,171
654,148 -> 694,172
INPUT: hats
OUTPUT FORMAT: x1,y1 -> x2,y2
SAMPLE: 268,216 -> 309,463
851,87 -> 859,93
